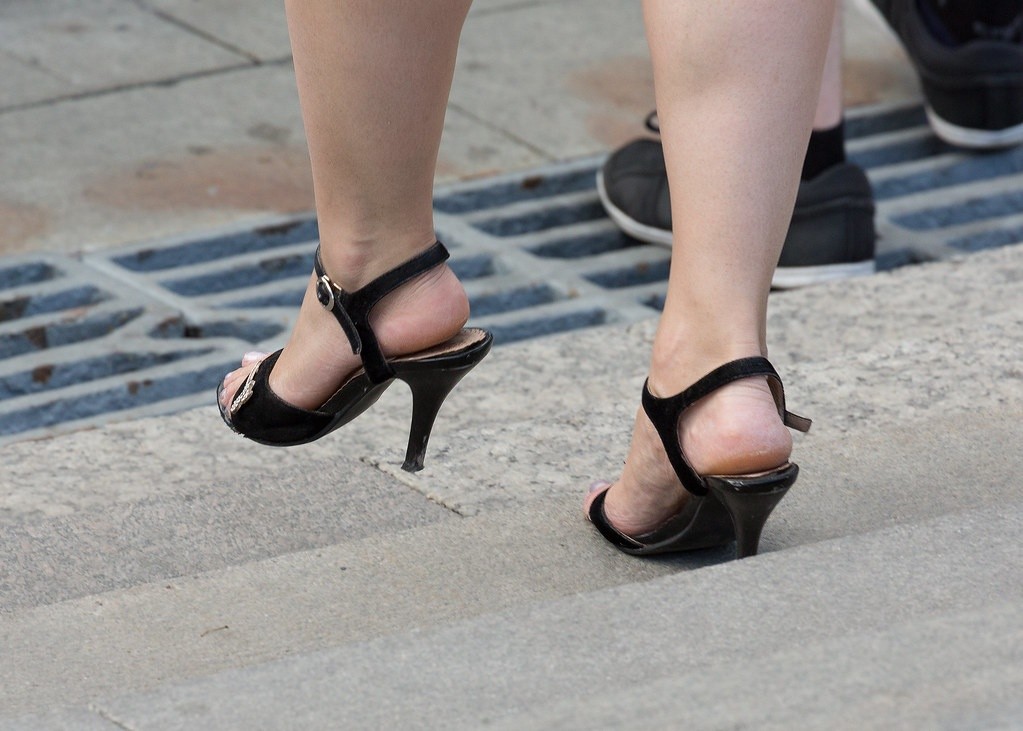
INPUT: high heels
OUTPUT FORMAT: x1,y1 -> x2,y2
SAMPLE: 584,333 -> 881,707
582,356 -> 812,560
216,240 -> 494,473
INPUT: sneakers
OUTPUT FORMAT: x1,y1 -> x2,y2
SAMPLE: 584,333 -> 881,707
855,0 -> 1023,149
596,110 -> 875,290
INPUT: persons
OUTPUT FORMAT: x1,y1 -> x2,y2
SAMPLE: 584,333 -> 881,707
596,1 -> 1023,288
216,0 -> 840,559
